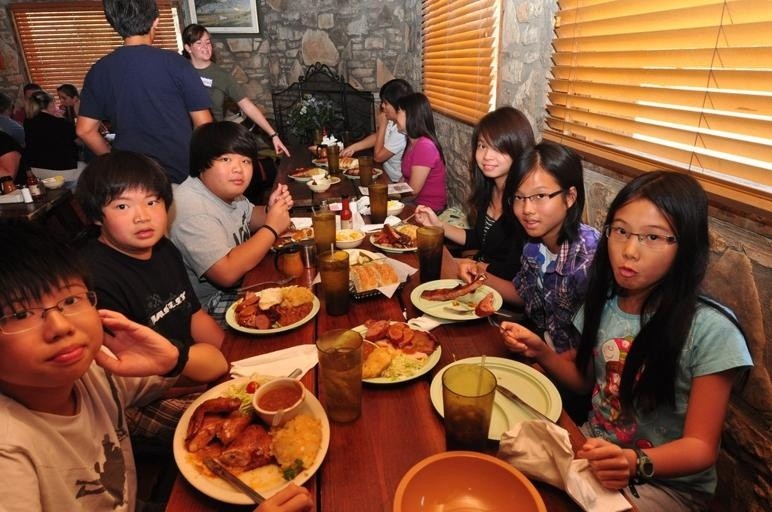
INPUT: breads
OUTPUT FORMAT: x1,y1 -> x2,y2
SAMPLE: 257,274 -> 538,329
339,158 -> 361,170
352,262 -> 398,293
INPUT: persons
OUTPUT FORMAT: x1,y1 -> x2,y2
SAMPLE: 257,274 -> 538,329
1,83 -> 108,185
1,219 -> 314,511
339,78 -> 413,184
414,106 -> 538,323
499,170 -> 755,511
75,150 -> 225,439
77,1 -> 214,213
395,92 -> 446,225
460,142 -> 601,376
165,120 -> 294,332
182,23 -> 290,156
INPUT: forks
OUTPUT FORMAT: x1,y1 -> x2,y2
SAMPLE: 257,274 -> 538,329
485,313 -> 510,339
395,206 -> 427,225
237,275 -> 295,293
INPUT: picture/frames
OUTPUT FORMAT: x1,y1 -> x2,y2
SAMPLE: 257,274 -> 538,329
187,0 -> 264,38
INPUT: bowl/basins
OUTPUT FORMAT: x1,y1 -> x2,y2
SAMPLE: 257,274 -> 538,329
333,229 -> 367,250
252,377 -> 306,430
392,450 -> 549,510
384,200 -> 405,216
42,175 -> 65,190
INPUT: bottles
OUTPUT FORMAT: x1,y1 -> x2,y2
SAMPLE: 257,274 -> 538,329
65,105 -> 74,120
25,167 -> 42,200
340,194 -> 354,231
0,176 -> 16,195
315,126 -> 328,142
319,199 -> 329,211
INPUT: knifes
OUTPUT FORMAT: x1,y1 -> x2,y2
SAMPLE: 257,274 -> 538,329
394,289 -> 408,322
495,384 -> 558,426
199,452 -> 266,506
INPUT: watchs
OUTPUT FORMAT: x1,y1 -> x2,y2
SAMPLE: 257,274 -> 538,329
632,447 -> 654,485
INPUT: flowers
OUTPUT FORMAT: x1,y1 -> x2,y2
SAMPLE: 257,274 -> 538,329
285,94 -> 344,136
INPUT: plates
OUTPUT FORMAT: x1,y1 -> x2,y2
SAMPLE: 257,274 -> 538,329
410,278 -> 505,321
369,224 -> 421,253
225,288 -> 321,335
174,374 -> 332,507
340,248 -> 382,268
340,320 -> 442,386
429,356 -> 564,443
269,216 -> 313,252
289,140 -> 384,193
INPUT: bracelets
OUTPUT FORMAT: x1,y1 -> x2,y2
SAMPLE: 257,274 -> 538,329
264,205 -> 269,216
271,132 -> 278,137
161,339 -> 193,378
263,224 -> 278,240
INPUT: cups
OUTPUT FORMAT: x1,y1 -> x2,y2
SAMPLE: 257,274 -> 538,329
315,328 -> 364,424
272,242 -> 304,280
415,225 -> 445,283
440,363 -> 497,453
298,238 -> 319,269
317,249 -> 351,316
311,210 -> 336,252
368,183 -> 390,224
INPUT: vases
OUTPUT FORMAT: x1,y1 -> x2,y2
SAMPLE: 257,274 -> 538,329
313,131 -> 327,147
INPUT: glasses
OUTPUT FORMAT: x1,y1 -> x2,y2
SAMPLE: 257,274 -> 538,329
604,223 -> 678,245
1,290 -> 97,335
508,189 -> 565,206
